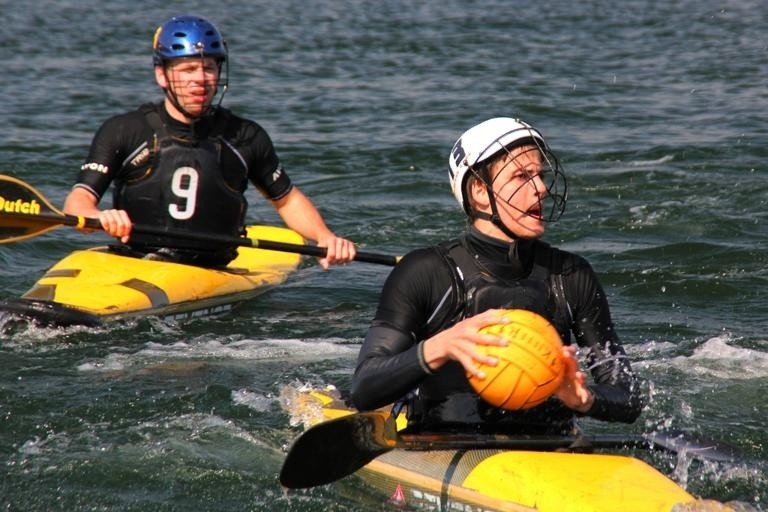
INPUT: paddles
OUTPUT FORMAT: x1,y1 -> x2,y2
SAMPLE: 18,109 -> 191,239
0,176 -> 403,266
280,411 -> 744,488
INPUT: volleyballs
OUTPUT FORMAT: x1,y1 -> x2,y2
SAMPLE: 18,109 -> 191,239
466,310 -> 563,411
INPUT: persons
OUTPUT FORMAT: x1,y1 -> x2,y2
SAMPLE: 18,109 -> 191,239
352,115 -> 643,453
63,16 -> 357,272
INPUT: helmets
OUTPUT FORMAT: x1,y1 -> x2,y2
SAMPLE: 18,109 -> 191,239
446,116 -> 567,223
151,15 -> 229,118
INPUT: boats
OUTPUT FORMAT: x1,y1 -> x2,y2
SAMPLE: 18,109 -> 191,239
285,381 -> 730,512
1,226 -> 304,325
